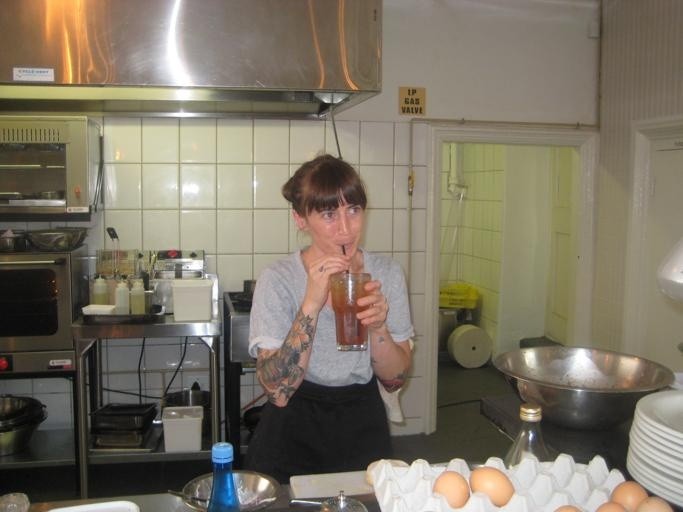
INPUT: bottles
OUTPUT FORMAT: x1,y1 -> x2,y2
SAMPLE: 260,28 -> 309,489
504,402 -> 552,470
207,441 -> 239,512
93,274 -> 145,314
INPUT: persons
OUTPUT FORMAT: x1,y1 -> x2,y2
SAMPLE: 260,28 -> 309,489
240,153 -> 416,485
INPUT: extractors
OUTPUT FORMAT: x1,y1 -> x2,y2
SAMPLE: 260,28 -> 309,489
0,1 -> 382,120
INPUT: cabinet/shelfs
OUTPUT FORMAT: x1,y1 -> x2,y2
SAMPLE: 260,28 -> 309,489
0,318 -> 222,499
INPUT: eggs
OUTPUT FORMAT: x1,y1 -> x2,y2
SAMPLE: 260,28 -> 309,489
433,471 -> 470,507
596,481 -> 673,512
470,467 -> 516,507
554,505 -> 581,512
365,459 -> 410,486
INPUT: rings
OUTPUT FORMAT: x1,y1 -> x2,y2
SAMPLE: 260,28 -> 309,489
318,265 -> 327,274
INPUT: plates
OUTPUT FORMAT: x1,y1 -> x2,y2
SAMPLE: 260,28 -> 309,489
181,470 -> 282,512
626,389 -> 682,509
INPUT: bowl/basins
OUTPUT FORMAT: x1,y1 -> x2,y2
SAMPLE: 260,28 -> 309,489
0,227 -> 90,252
494,346 -> 675,431
0,394 -> 49,457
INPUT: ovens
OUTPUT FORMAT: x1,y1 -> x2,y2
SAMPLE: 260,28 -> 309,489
0,115 -> 104,214
0,243 -> 90,375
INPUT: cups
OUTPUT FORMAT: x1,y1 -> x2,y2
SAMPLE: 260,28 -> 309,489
329,273 -> 371,351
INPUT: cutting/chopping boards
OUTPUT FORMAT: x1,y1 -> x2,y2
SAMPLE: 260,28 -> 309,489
289,471 -> 377,500
